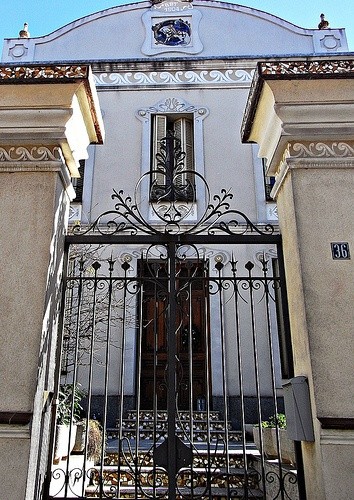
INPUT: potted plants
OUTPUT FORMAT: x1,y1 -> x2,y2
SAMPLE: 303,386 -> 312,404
253,412 -> 298,470
51,382 -> 85,465
